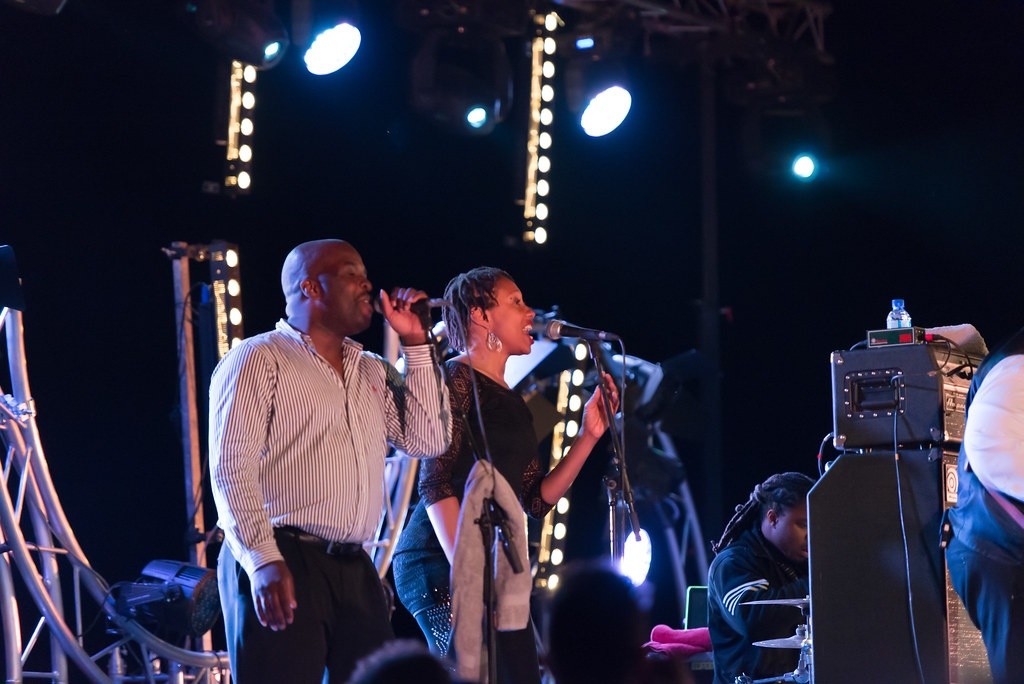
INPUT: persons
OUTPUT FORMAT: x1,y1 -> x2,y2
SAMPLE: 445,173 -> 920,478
701,469 -> 819,682
393,266 -> 620,681
204,236 -> 456,684
347,638 -> 453,684
946,339 -> 1024,683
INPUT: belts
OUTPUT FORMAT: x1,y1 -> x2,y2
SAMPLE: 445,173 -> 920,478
274,525 -> 362,555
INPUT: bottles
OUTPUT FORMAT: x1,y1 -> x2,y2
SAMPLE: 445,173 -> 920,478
887,299 -> 912,329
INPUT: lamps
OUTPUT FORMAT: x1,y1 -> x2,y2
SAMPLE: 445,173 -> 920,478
216,10 -> 285,71
288,3 -> 366,75
518,9 -> 565,245
220,54 -> 259,192
118,559 -> 228,641
568,65 -> 636,142
530,336 -> 588,599
166,239 -> 245,576
599,525 -> 658,588
454,90 -> 497,131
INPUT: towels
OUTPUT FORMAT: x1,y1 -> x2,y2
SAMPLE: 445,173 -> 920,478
643,623 -> 715,660
444,457 -> 535,684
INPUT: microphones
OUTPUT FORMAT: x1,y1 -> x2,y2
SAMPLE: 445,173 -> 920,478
373,296 -> 448,313
546,319 -> 619,342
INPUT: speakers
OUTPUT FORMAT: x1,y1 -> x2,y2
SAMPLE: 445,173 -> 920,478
806,345 -> 993,684
